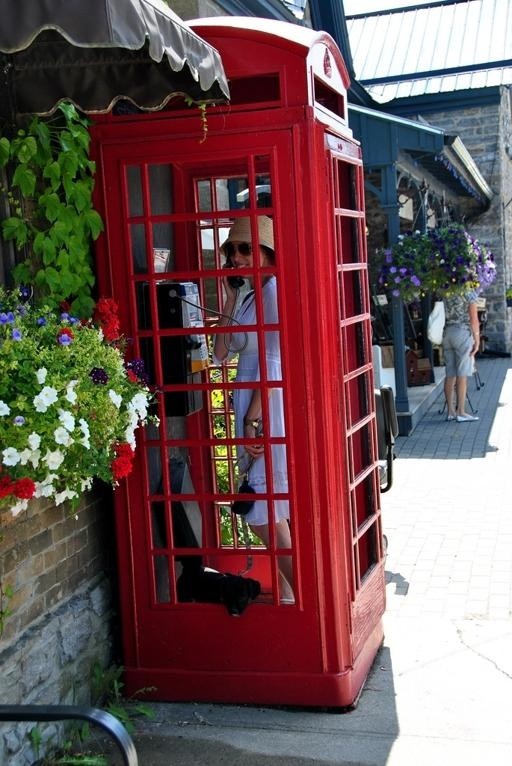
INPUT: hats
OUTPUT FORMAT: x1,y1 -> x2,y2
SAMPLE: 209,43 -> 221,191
220,215 -> 275,251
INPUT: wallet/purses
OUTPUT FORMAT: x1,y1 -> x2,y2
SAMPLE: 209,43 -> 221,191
232,483 -> 255,515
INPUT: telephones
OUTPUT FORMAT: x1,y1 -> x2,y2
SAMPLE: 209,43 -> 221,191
226,255 -> 245,289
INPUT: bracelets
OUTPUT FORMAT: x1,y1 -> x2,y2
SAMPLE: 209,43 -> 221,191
244,414 -> 263,434
473,331 -> 480,336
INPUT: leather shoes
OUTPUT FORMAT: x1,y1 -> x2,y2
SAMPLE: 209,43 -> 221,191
446,415 -> 456,421
456,416 -> 479,421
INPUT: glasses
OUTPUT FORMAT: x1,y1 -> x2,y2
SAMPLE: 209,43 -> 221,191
222,242 -> 253,257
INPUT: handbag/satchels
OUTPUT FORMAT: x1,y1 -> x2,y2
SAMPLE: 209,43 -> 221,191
427,302 -> 445,344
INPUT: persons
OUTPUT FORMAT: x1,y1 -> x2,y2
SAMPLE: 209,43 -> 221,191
437,282 -> 482,421
207,216 -> 304,600
